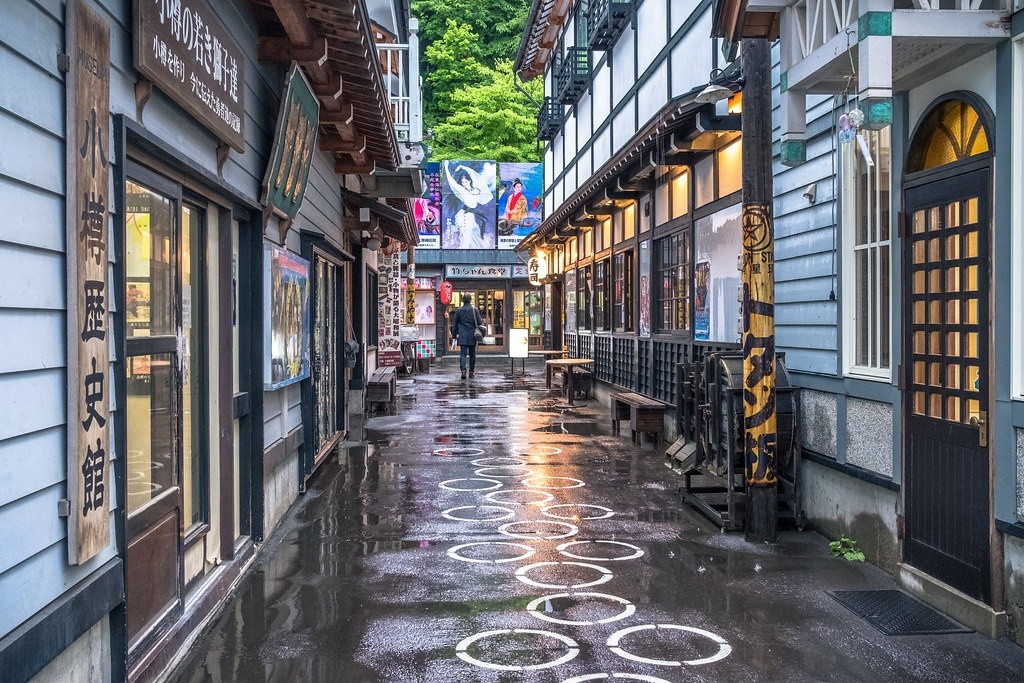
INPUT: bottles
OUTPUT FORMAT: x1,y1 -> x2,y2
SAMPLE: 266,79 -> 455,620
452,339 -> 457,351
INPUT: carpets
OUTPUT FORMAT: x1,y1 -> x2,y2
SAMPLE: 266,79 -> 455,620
823,588 -> 977,636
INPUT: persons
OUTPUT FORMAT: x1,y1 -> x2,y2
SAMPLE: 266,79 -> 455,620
421,306 -> 434,322
452,294 -> 483,379
447,305 -> 456,345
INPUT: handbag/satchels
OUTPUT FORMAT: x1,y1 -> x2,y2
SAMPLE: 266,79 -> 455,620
474,325 -> 487,342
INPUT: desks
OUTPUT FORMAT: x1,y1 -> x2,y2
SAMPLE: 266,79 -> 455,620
547,359 -> 594,409
528,350 -> 570,391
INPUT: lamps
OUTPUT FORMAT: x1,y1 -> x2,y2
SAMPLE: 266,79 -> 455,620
694,67 -> 746,105
367,230 -> 382,251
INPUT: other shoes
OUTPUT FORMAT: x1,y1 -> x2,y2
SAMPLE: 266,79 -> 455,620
461,371 -> 466,379
469,373 -> 475,378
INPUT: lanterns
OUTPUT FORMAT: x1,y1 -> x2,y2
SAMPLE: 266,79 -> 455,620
528,257 -> 546,286
440,282 -> 453,306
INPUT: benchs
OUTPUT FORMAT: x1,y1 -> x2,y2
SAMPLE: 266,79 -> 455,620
368,372 -> 396,412
610,392 -> 666,448
372,366 -> 397,388
562,367 -> 592,401
